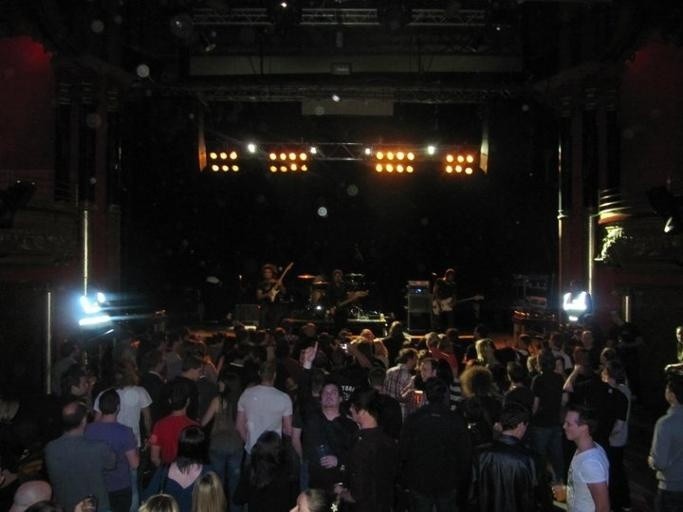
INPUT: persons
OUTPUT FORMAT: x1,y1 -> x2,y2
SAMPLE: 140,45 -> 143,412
330,268 -> 359,330
432,267 -> 457,332
256,263 -> 286,329
306,288 -> 336,320
1,311 -> 683,511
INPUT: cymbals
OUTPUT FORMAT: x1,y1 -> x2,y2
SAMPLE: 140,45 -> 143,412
297,275 -> 316,280
313,282 -> 330,286
345,273 -> 363,276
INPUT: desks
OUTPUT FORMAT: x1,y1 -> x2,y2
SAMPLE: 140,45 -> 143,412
347,313 -> 386,336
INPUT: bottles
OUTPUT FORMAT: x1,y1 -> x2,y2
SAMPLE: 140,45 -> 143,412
320,445 -> 325,459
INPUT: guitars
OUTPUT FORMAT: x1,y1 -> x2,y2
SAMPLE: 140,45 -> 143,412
433,296 -> 485,315
325,293 -> 361,320
265,261 -> 294,302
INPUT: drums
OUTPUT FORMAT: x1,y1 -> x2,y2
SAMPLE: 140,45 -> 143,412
312,290 -> 326,304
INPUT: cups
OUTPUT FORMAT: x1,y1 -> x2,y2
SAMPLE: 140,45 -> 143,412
547,478 -> 565,502
379,313 -> 384,320
415,390 -> 423,405
